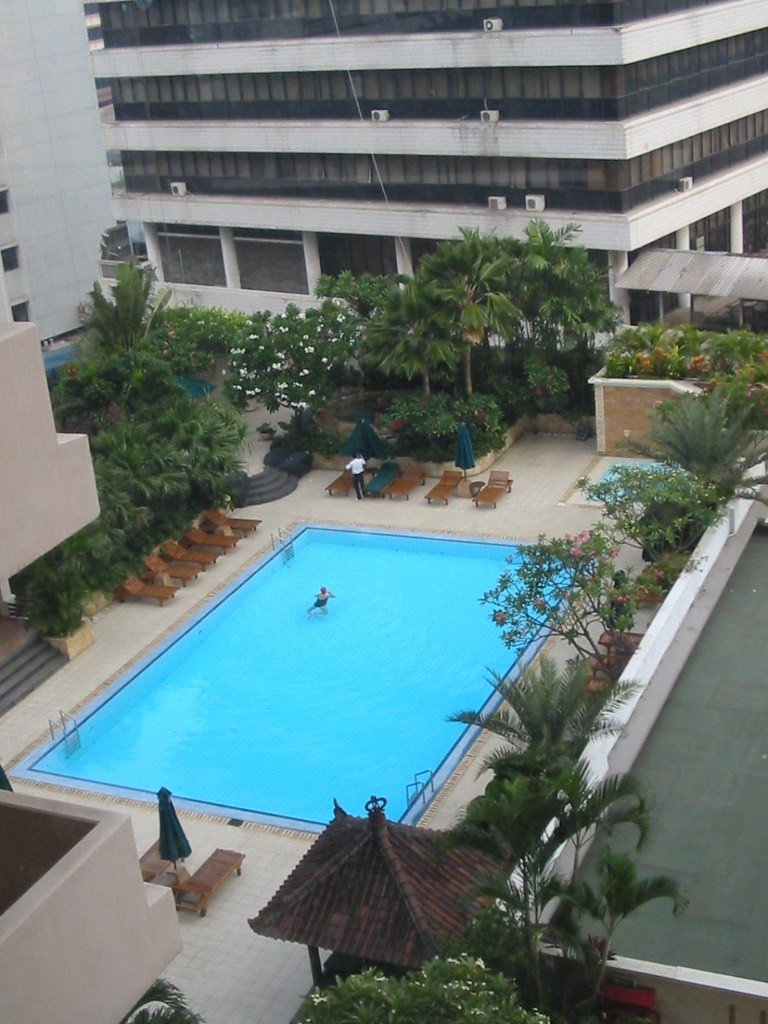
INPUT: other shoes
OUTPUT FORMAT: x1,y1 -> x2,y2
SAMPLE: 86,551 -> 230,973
355,497 -> 361,501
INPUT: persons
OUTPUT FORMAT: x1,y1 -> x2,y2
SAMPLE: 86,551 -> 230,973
307,587 -> 335,619
345,450 -> 368,499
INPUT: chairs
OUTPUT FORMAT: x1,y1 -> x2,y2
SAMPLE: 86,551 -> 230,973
584,678 -> 615,705
162,540 -> 220,572
628,566 -> 679,609
598,630 -> 645,655
588,653 -> 631,677
363,462 -> 399,498
381,466 -> 427,500
473,471 -> 513,508
202,507 -> 262,538
116,571 -> 180,607
425,471 -> 463,505
142,552 -> 202,587
140,839 -> 185,882
168,848 -> 246,917
184,527 -> 241,555
325,462 -> 354,497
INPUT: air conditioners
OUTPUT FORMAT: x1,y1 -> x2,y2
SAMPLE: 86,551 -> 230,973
371,110 -> 389,122
525,194 -> 545,212
678,176 -> 693,192
481,110 -> 499,124
171,182 -> 187,196
484,19 -> 502,32
488,196 -> 506,210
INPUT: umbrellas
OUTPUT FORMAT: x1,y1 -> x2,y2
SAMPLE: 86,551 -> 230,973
456,422 -> 476,482
339,418 -> 387,469
157,786 -> 192,871
174,375 -> 214,400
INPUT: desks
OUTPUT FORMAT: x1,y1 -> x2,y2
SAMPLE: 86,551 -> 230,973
365,468 -> 378,478
474,481 -> 485,491
202,521 -> 217,535
152,874 -> 176,886
141,572 -> 155,583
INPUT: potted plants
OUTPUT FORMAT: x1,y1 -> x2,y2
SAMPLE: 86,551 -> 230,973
256,423 -> 277,439
